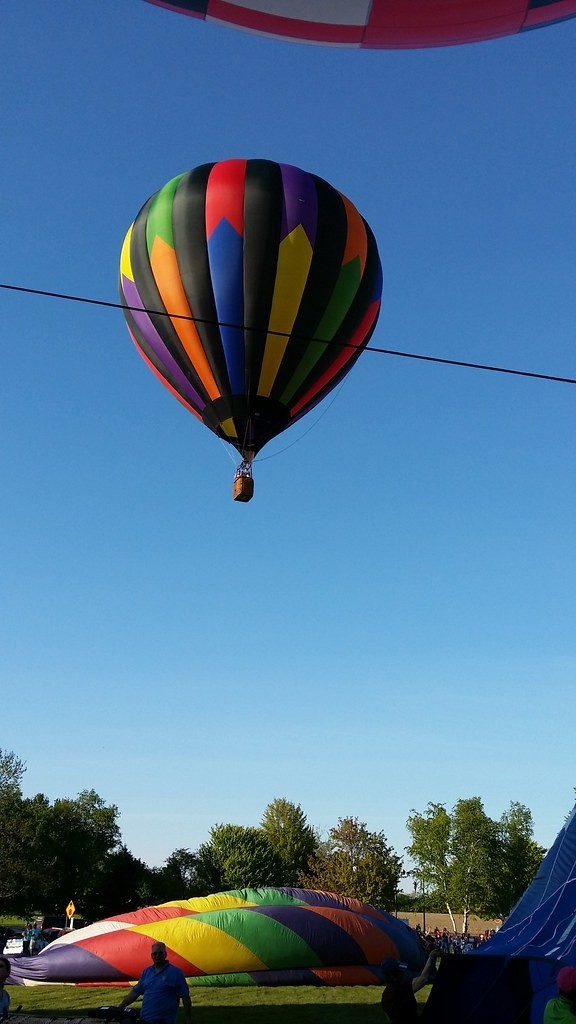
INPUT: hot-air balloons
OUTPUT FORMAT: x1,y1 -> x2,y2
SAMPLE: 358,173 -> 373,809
117,157 -> 385,502
6,886 -> 436,988
132,0 -> 576,51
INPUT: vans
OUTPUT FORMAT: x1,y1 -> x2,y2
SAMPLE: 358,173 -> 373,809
35,916 -> 67,930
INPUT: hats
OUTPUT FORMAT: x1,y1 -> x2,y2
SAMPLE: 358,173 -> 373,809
28,924 -> 31,926
381,958 -> 409,975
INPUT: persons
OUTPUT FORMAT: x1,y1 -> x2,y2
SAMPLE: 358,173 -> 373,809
117,942 -> 192,1023
22,922 -> 41,955
0,957 -> 11,1013
381,949 -> 439,1024
416,924 -> 496,953
543,967 -> 576,1024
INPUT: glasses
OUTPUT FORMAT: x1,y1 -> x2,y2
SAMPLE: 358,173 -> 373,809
152,951 -> 166,955
